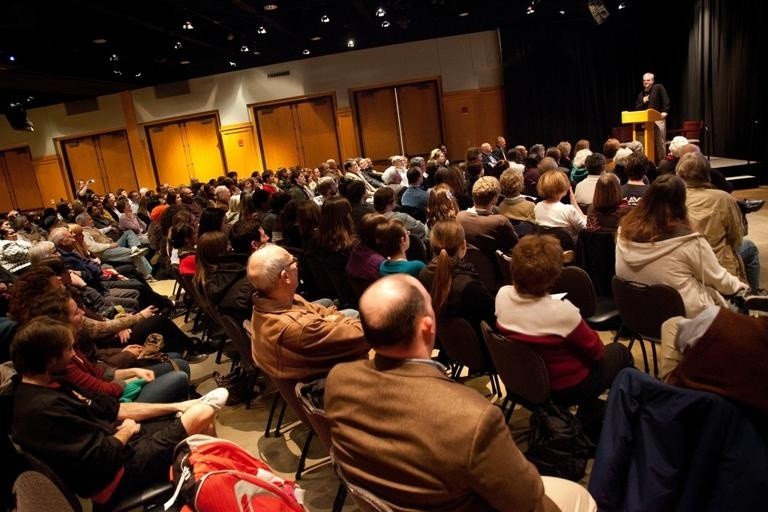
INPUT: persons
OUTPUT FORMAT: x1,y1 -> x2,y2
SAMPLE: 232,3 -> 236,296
635,72 -> 670,167
1,133 -> 767,510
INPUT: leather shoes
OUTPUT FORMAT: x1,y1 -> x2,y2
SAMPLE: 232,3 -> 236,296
745,197 -> 765,214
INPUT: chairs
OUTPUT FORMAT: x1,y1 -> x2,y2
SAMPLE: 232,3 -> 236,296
666,120 -> 705,153
612,127 -> 634,143
0,183 -> 767,512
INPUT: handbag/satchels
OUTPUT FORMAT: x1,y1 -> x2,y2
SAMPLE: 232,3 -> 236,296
211,363 -> 249,407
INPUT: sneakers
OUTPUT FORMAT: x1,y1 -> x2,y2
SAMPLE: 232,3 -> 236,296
180,349 -> 211,365
189,335 -> 218,353
128,246 -> 149,259
201,385 -> 230,411
169,307 -> 188,319
144,275 -> 158,282
741,287 -> 768,313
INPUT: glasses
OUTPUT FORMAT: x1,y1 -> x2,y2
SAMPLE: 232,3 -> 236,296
277,255 -> 299,281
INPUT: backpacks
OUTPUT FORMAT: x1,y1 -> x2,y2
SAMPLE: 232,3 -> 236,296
163,433 -> 310,512
523,398 -> 593,482
